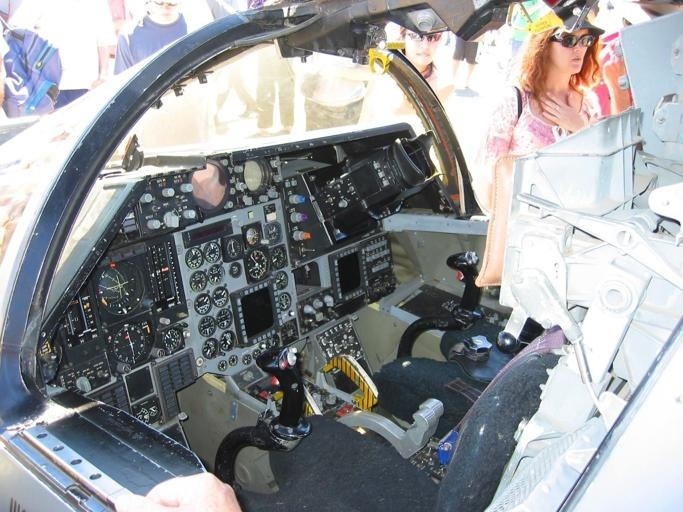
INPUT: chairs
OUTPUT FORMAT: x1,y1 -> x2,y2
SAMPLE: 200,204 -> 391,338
233,319 -> 566,512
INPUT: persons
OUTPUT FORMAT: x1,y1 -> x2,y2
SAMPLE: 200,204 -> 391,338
481,7 -> 606,213
449,36 -> 478,95
112,0 -> 189,78
299,44 -> 371,135
508,0 -> 549,60
112,470 -> 244,512
396,24 -> 455,106
250,45 -> 298,142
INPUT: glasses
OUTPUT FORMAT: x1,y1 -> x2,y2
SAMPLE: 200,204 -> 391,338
407,33 -> 441,42
548,33 -> 593,47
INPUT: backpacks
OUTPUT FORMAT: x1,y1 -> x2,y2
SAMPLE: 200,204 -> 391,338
0,17 -> 62,118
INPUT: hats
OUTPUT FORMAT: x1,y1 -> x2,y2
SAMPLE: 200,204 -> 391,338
528,11 -> 605,37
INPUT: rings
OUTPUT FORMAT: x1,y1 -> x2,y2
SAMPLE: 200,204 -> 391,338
554,104 -> 559,111
